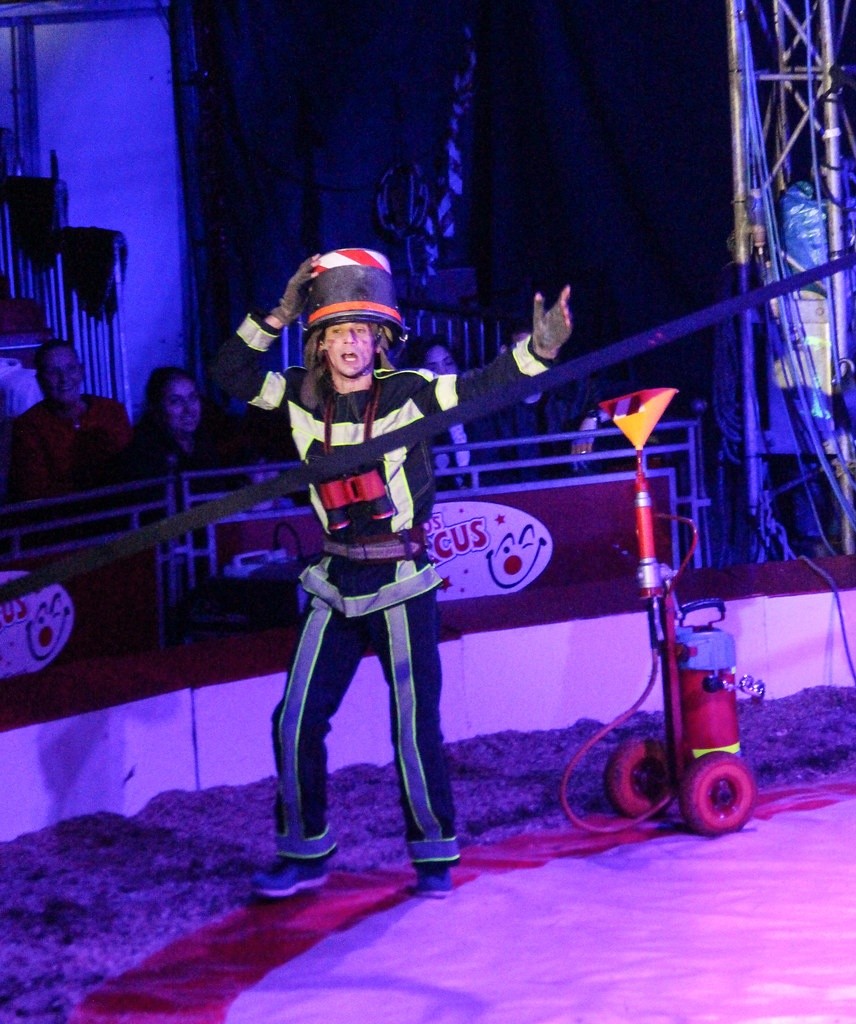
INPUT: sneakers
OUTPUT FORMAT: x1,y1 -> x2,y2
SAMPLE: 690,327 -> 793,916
250,862 -> 330,898
415,865 -> 451,898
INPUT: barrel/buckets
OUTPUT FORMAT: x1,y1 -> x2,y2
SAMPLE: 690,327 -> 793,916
298,259 -> 411,358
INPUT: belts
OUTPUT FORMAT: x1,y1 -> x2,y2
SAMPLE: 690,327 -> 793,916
322,540 -> 420,563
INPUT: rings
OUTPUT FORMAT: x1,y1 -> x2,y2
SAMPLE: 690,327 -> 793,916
581,451 -> 586,454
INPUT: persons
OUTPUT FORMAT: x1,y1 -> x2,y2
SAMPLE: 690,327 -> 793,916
410,340 -> 518,491
490,330 -> 610,482
216,247 -> 572,897
100,367 -> 253,532
8,339 -> 132,536
